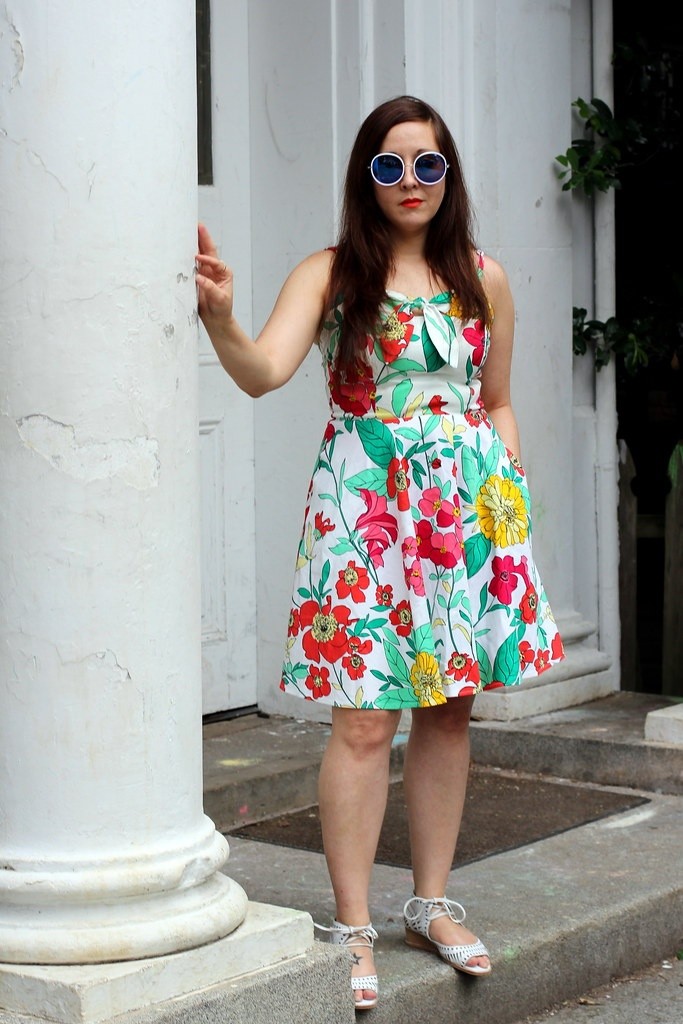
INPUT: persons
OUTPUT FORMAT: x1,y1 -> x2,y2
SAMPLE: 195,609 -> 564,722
195,94 -> 564,1008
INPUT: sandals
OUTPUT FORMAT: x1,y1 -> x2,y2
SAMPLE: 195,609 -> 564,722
402,889 -> 492,976
313,918 -> 379,1010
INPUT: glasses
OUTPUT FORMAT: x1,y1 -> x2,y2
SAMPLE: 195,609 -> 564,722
366,150 -> 449,187
